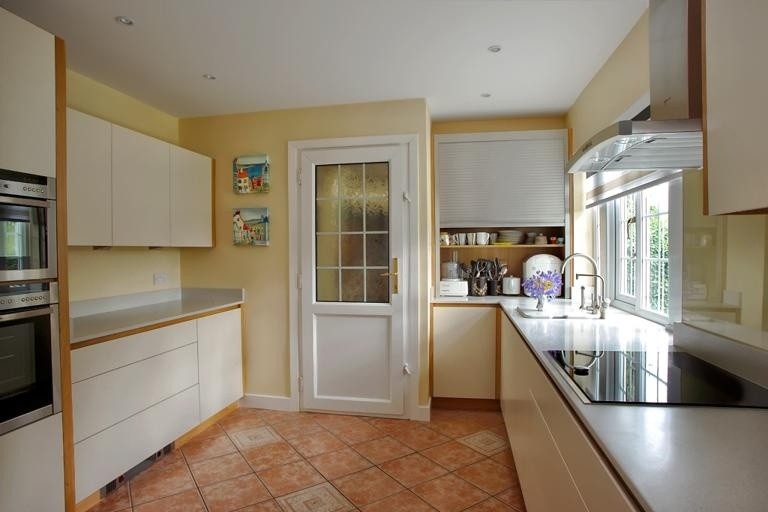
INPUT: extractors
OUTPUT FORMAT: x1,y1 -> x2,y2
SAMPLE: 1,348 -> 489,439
562,1 -> 703,172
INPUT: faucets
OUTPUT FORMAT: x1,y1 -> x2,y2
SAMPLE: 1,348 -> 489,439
575,272 -> 610,319
560,253 -> 601,315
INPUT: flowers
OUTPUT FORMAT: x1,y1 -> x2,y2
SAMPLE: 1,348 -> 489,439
521,268 -> 564,303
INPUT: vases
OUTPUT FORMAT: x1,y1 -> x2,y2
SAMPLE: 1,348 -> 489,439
535,294 -> 546,312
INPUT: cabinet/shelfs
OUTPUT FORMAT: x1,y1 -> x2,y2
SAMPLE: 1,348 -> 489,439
67,107 -> 213,251
700,0 -> 766,216
500,309 -> 644,512
436,223 -> 570,297
70,316 -> 198,503
431,304 -> 497,401
197,307 -> 244,425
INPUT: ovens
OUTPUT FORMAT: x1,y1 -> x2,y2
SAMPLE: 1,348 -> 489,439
0,193 -> 61,438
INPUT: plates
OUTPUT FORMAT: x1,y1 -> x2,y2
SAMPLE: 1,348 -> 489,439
498,230 -> 524,243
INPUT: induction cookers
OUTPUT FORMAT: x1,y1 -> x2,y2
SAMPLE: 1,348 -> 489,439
543,349 -> 767,409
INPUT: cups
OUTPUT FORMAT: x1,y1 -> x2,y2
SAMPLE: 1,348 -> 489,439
457,232 -> 466,245
476,232 -> 489,245
466,233 -> 476,245
